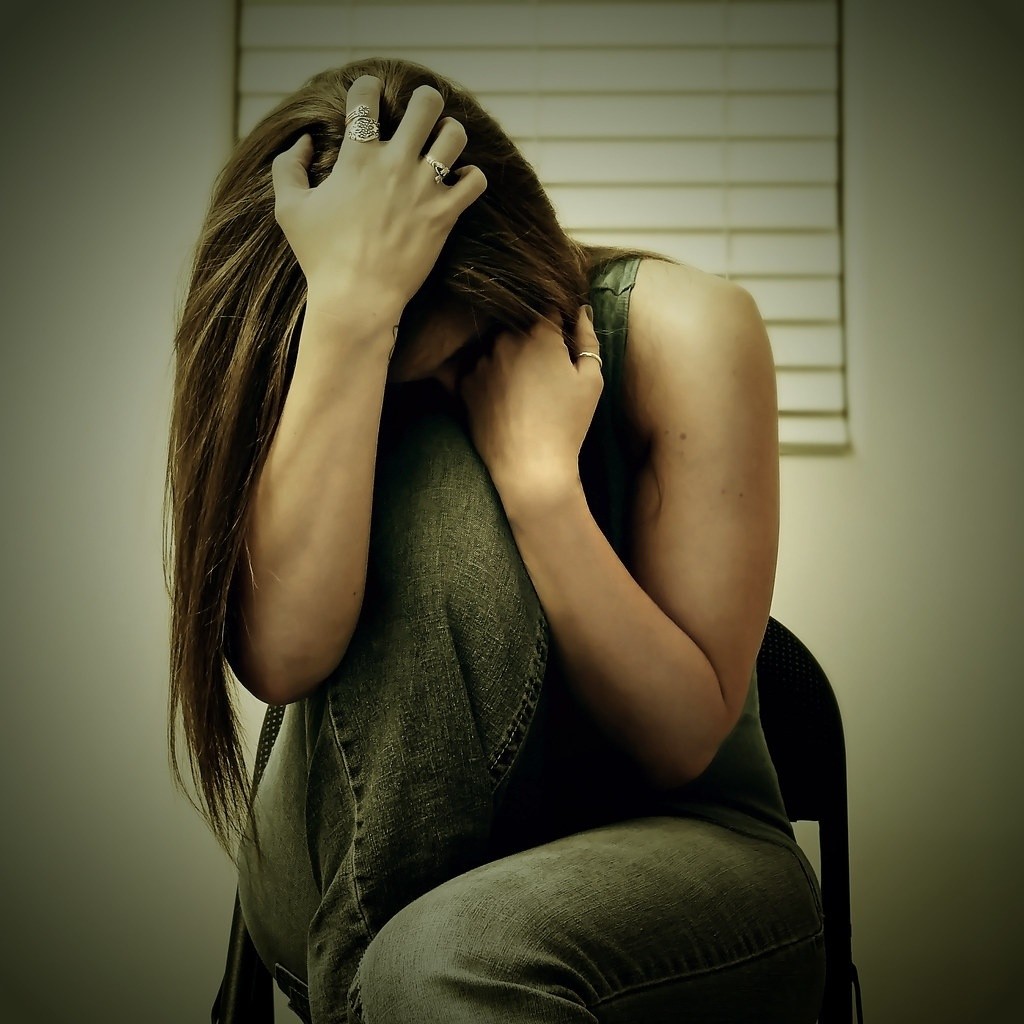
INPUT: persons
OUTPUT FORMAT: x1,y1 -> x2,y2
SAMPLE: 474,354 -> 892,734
164,55 -> 828,1024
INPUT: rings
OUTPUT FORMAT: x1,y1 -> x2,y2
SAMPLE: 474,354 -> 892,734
344,106 -> 380,143
577,352 -> 602,368
423,154 -> 450,183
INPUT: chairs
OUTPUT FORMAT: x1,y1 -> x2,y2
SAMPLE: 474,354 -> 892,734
211,614 -> 869,1024
